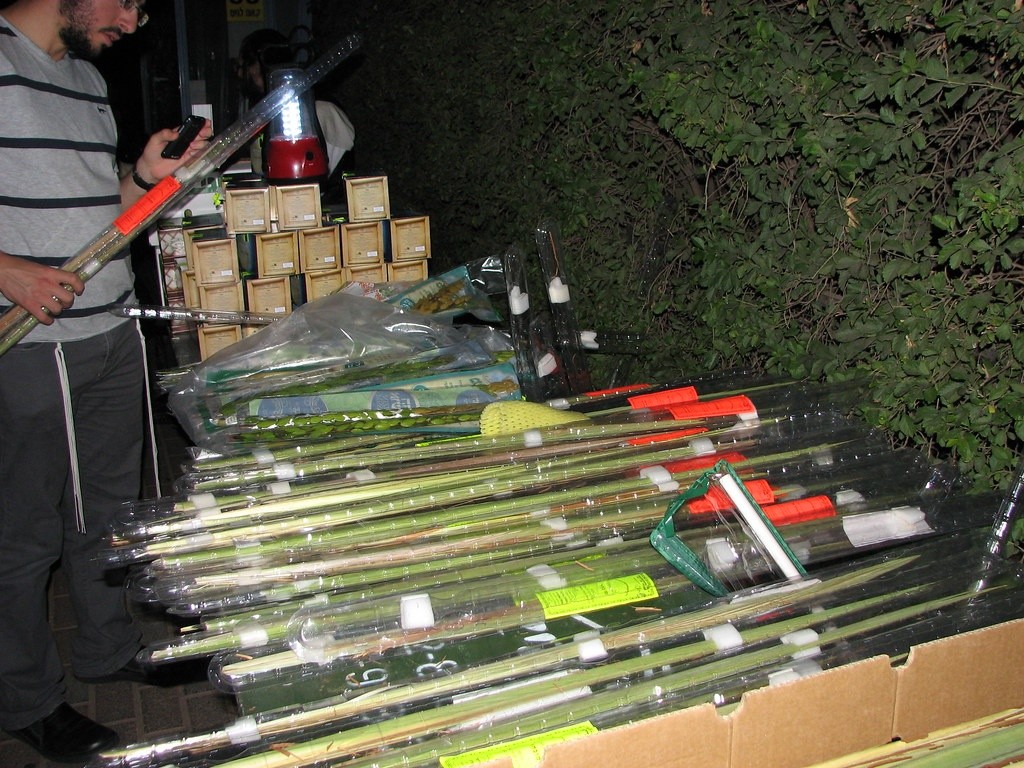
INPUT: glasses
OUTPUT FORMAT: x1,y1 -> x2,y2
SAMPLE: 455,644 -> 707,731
117,0 -> 149,28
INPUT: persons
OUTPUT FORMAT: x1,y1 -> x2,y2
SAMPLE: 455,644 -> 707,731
0,0 -> 212,768
239,29 -> 294,110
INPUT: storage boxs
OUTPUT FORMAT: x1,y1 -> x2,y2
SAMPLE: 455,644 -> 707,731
174,260 -> 200,308
347,263 -> 387,284
345,174 -> 391,223
193,229 -> 239,284
226,181 -> 272,234
275,179 -> 322,231
298,219 -> 341,274
386,211 -> 432,263
244,275 -> 292,315
387,260 -> 429,283
254,230 -> 301,279
328,212 -> 385,268
182,213 -> 225,271
302,269 -> 346,304
199,280 -> 245,328
197,323 -> 242,361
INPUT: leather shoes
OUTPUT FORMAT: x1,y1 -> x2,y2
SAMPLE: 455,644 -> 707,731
5,698 -> 121,766
71,643 -> 195,686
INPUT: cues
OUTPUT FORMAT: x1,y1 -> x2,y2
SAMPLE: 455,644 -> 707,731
1,29 -> 368,361
92,366 -> 1023,767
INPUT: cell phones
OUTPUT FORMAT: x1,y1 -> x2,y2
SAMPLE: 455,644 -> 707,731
160,114 -> 205,160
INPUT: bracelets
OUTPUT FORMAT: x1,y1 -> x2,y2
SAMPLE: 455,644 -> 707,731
132,162 -> 156,191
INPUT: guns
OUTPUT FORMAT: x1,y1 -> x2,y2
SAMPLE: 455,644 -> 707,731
504,223 -> 647,403
